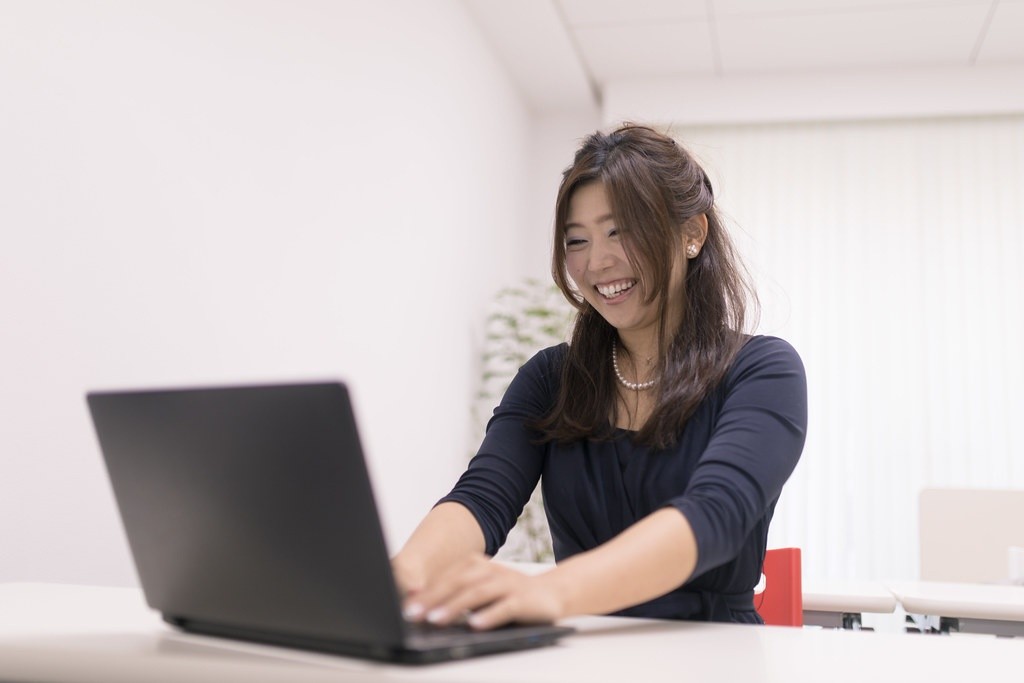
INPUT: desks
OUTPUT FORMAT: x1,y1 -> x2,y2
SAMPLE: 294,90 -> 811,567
0,577 -> 1024,683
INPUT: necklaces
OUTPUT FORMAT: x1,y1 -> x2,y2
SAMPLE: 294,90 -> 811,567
611,339 -> 661,391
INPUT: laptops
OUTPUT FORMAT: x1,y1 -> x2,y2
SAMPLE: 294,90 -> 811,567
85,382 -> 574,666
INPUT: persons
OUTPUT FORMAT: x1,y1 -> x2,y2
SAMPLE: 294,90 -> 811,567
387,124 -> 808,627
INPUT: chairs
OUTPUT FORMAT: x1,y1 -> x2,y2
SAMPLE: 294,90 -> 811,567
902,488 -> 1024,638
766,473 -> 897,631
